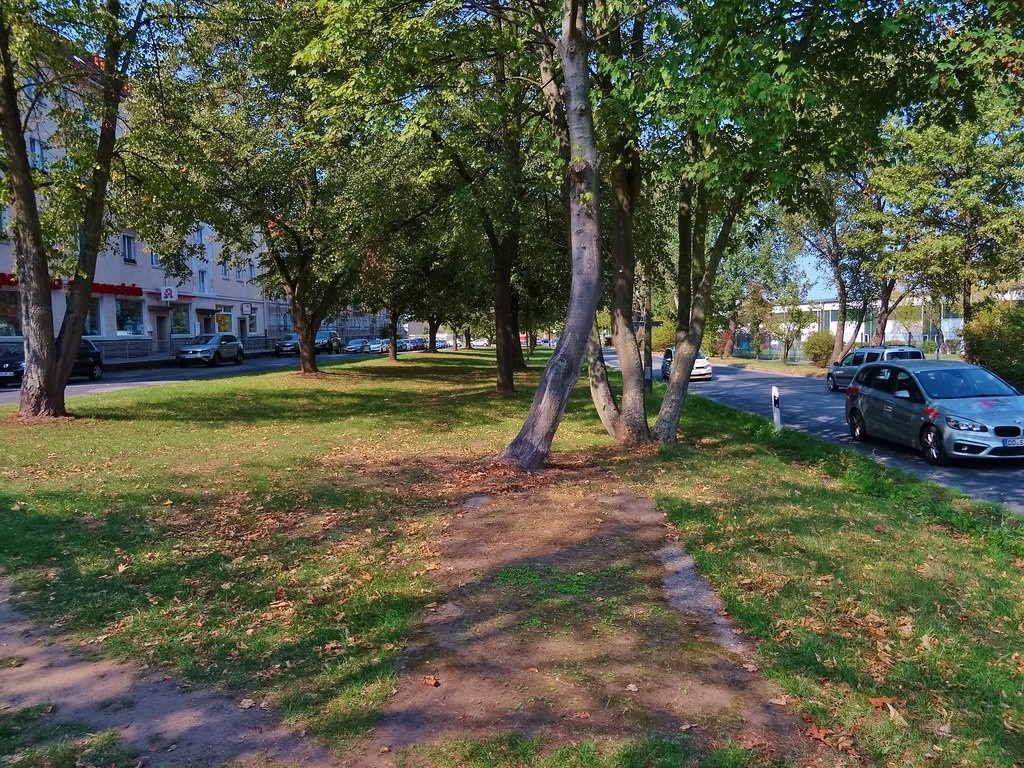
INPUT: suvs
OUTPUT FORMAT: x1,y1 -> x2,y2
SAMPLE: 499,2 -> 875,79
826,344 -> 927,391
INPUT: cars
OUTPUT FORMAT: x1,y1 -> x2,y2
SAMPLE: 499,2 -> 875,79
275,333 -> 300,357
0,337 -> 104,386
314,331 -> 342,355
844,358 -> 1024,467
470,339 -> 488,347
178,333 -> 245,368
660,348 -> 712,381
342,338 -> 371,354
369,337 -> 463,354
